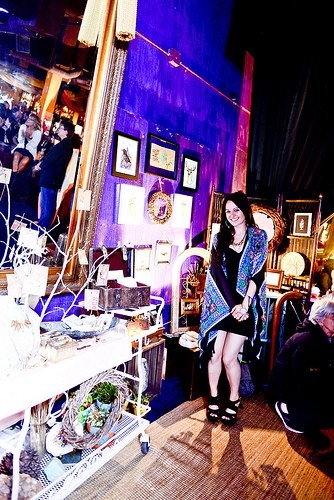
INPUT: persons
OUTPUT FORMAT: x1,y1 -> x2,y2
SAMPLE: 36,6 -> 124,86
0,101 -> 41,192
197,190 -> 269,428
33,118 -> 76,236
270,292 -> 334,450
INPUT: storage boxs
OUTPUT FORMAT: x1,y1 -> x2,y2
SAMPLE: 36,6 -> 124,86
91,281 -> 150,310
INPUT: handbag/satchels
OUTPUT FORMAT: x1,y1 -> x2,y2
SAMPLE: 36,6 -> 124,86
0,162 -> 12,184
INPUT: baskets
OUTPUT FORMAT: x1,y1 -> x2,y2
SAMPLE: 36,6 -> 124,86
62,370 -> 132,450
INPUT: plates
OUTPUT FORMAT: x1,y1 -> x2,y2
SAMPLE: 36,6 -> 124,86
40,317 -> 119,338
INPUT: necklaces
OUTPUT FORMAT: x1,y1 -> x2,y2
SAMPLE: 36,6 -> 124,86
234,233 -> 246,246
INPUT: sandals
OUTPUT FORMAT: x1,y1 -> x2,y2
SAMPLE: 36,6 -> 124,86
221,397 -> 241,426
207,395 -> 221,422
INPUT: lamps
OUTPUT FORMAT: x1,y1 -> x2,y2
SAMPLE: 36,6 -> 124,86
168,48 -> 181,68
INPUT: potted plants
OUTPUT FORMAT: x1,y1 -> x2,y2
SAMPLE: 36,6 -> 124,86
69,382 -> 118,447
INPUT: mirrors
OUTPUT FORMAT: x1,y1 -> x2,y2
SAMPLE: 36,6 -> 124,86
0,0 -> 129,296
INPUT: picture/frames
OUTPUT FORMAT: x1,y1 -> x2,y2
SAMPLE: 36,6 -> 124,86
293,213 -> 312,237
155,240 -> 171,263
180,154 -> 200,193
134,245 -> 152,270
265,268 -> 284,289
181,299 -> 199,314
144,132 -> 181,180
112,130 -> 141,181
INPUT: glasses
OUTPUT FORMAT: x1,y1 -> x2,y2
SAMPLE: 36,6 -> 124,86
28,118 -> 34,121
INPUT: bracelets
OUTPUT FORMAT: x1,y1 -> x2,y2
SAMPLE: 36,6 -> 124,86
239,304 -> 248,315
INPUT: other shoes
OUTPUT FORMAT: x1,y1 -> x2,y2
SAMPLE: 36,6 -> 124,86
275,402 -> 303,434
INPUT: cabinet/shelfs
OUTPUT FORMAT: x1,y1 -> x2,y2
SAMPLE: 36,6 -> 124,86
180,188 -> 322,400
0,295 -> 165,500
